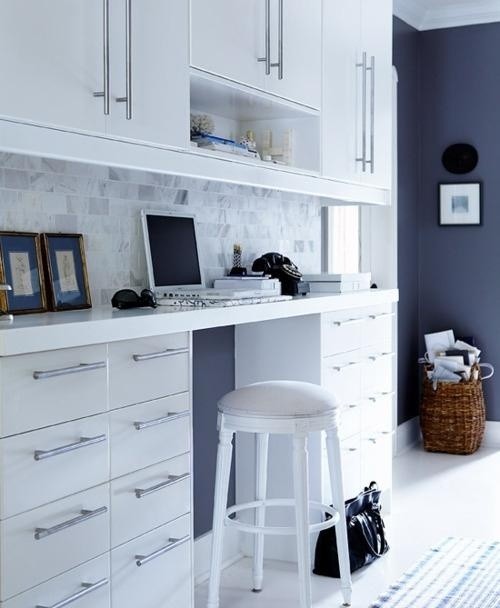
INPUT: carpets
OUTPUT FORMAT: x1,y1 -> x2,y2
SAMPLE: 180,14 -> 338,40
369,535 -> 500,608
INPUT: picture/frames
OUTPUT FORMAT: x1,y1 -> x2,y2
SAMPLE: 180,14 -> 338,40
0,230 -> 48,314
437,180 -> 483,227
41,232 -> 93,308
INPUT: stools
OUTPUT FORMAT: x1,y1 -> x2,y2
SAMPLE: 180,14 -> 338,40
205,379 -> 353,607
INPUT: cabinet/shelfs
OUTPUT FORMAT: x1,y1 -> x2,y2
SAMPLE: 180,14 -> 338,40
189,1 -> 321,119
186,72 -> 322,196
2,1 -> 192,150
1,330 -> 195,608
234,302 -> 400,564
318,1 -> 395,206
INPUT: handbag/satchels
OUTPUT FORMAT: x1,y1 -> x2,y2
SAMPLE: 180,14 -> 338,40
312,481 -> 390,579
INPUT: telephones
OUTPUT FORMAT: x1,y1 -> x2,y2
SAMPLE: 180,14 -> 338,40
254,253 -> 311,295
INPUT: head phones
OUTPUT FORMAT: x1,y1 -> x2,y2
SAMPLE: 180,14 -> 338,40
111,289 -> 157,310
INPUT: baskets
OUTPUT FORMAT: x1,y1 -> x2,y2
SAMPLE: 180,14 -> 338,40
419,365 -> 486,455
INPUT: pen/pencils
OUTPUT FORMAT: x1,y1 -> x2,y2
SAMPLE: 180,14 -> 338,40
233,242 -> 242,267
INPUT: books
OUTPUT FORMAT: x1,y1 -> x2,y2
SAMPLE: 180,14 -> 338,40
213,278 -> 280,290
309,281 -> 371,293
300,272 -> 372,283
424,328 -> 482,383
224,275 -> 269,280
192,133 -> 250,156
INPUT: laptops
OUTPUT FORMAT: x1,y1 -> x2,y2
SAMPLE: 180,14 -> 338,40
141,209 -> 279,299
